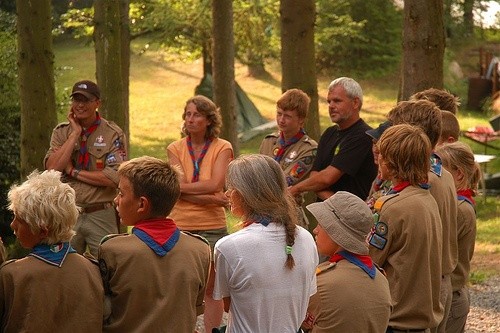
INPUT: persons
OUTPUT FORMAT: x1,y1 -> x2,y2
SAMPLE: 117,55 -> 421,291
0,168 -> 111,333
299,191 -> 392,333
289,76 -> 379,203
435,141 -> 480,333
166,95 -> 234,252
226,89 -> 319,206
388,100 -> 458,333
213,153 -> 319,333
43,80 -> 127,258
367,124 -> 444,333
98,157 -> 211,333
365,88 -> 459,214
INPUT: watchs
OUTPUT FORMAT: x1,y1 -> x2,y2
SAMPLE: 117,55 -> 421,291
74,169 -> 80,179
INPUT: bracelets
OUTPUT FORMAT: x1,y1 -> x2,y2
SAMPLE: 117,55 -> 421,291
69,168 -> 74,176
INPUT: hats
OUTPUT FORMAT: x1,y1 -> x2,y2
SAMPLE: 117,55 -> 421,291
70,80 -> 100,99
305,191 -> 374,255
365,121 -> 390,140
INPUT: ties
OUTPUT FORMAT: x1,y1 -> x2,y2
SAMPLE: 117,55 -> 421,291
327,250 -> 376,279
186,136 -> 212,183
386,180 -> 432,195
132,218 -> 180,257
29,241 -> 77,268
274,128 -> 306,164
78,111 -> 101,169
457,188 -> 476,214
429,153 -> 442,177
243,214 -> 272,227
373,174 -> 386,192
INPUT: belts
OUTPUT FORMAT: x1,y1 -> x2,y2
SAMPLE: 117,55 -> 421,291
388,328 -> 426,333
79,201 -> 112,213
